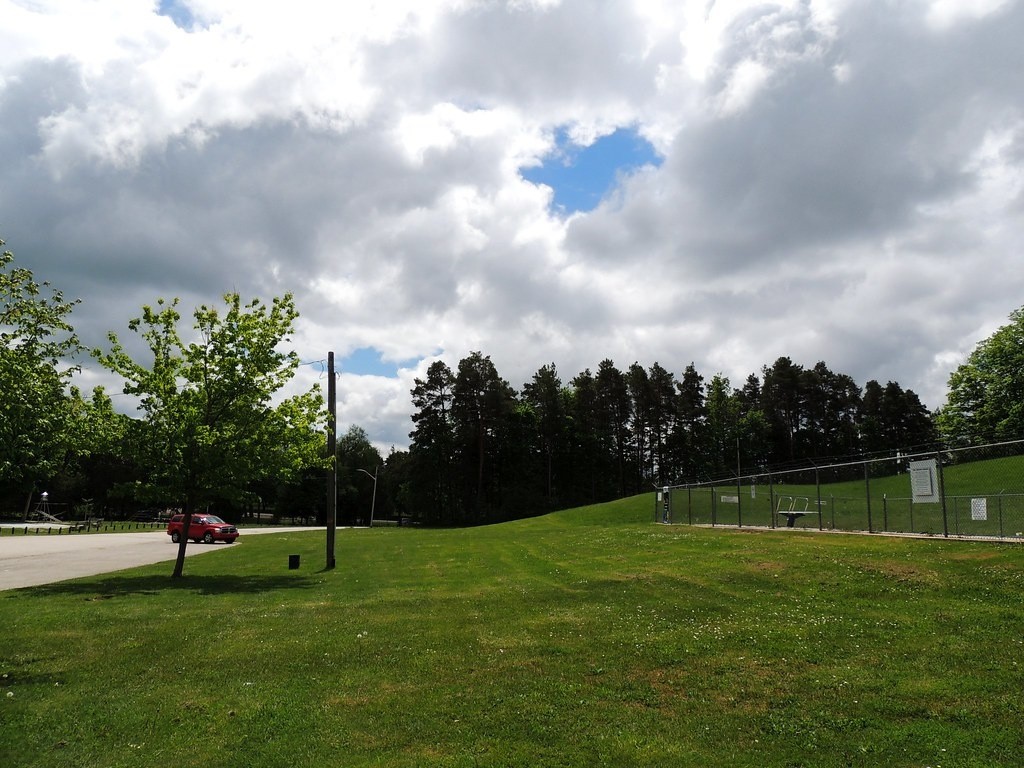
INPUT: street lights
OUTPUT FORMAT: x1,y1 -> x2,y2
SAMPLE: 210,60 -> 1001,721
357,465 -> 378,527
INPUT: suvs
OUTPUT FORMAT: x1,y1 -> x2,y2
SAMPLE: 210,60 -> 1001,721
167,514 -> 239,544
